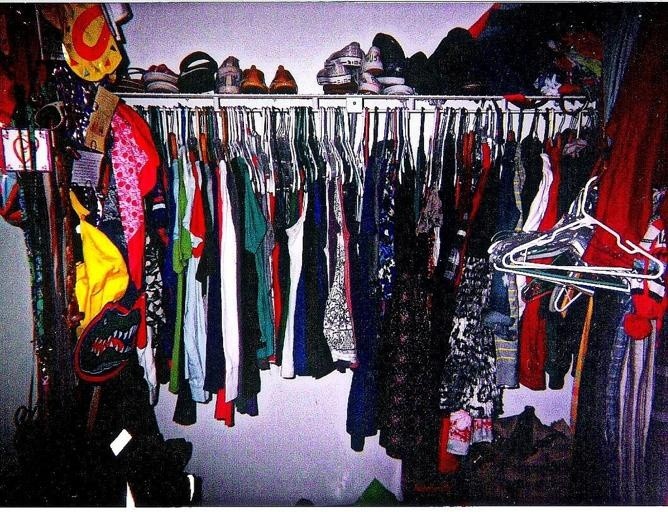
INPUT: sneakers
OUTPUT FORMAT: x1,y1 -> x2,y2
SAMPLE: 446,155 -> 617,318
357,72 -> 383,94
241,65 -> 268,93
214,55 -> 242,94
315,64 -> 352,86
360,45 -> 384,76
323,41 -> 362,67
375,59 -> 406,85
268,64 -> 298,93
381,83 -> 414,95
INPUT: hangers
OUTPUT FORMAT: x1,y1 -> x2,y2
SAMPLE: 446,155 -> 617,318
488,172 -> 665,314
